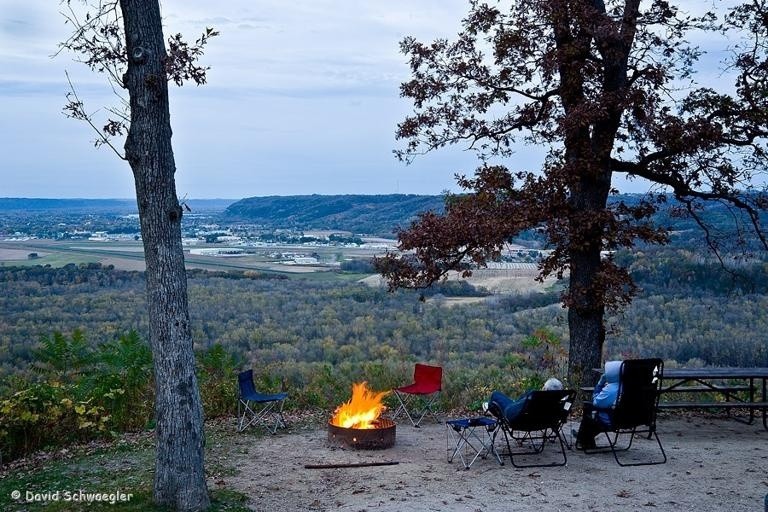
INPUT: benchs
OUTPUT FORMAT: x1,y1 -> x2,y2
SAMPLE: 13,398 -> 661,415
579,385 -> 758,393
655,401 -> 768,408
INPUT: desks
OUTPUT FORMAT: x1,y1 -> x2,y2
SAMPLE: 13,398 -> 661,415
592,367 -> 768,439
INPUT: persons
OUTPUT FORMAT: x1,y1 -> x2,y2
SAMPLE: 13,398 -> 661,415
481,376 -> 563,423
571,360 -> 625,450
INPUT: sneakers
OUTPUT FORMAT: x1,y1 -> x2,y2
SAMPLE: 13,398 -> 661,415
572,429 -> 578,438
483,403 -> 493,416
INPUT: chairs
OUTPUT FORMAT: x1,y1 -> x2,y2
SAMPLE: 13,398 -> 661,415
484,389 -> 577,468
391,363 -> 442,427
575,357 -> 666,466
446,417 -> 504,470
238,369 -> 288,435
